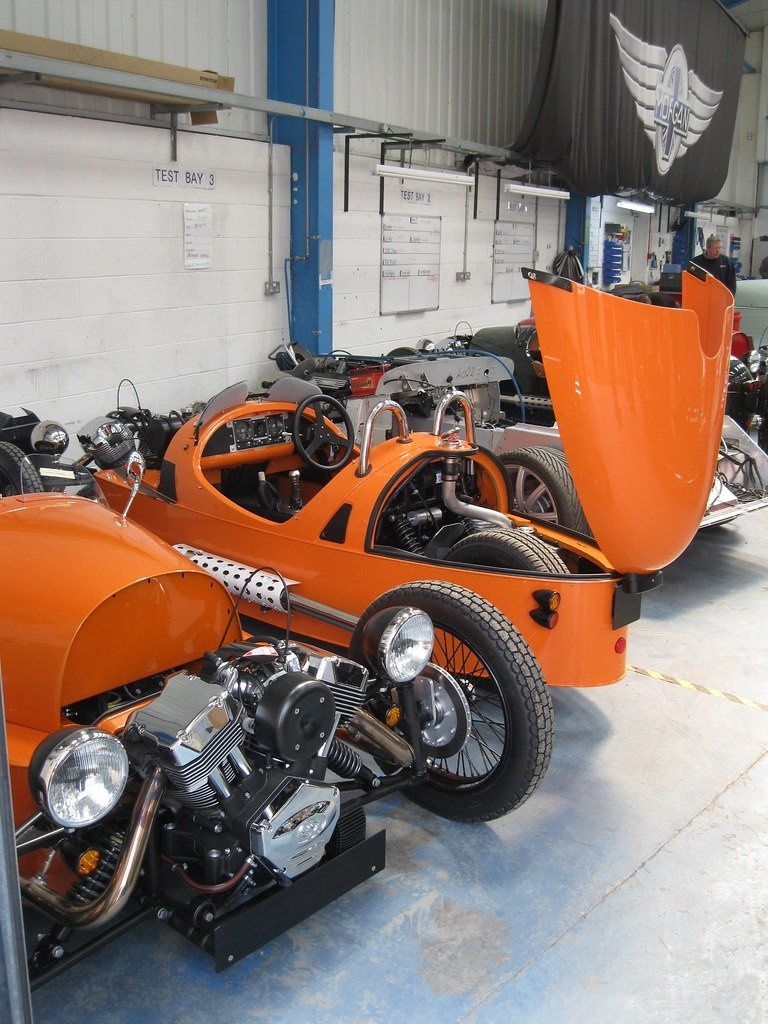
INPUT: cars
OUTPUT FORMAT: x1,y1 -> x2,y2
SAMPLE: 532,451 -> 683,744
0,259 -> 767,997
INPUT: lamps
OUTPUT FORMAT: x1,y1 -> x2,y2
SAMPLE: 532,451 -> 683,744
684,203 -> 711,219
616,191 -> 654,213
370,143 -> 474,186
504,161 -> 570,200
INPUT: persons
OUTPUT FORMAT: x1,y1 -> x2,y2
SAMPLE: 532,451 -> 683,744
759,254 -> 768,278
690,235 -> 736,299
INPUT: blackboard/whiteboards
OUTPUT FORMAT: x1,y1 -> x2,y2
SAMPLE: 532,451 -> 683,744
380,213 -> 442,315
491,220 -> 538,303
